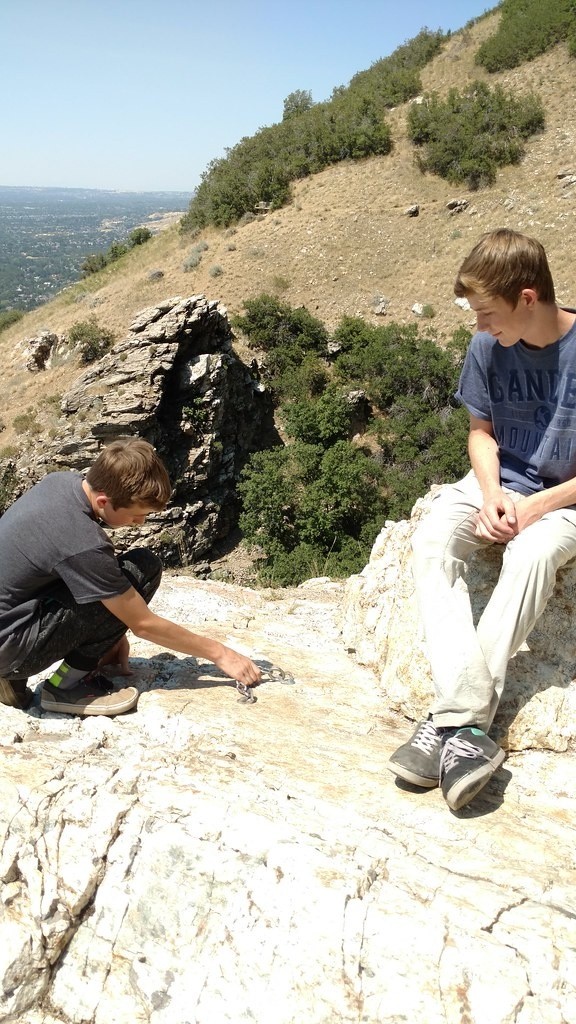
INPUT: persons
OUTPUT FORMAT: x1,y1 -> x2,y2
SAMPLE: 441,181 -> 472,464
0,439 -> 261,716
386,229 -> 576,810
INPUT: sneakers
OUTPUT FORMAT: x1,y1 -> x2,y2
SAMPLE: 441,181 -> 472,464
439,726 -> 506,811
40,679 -> 138,715
0,677 -> 33,709
386,719 -> 460,787
